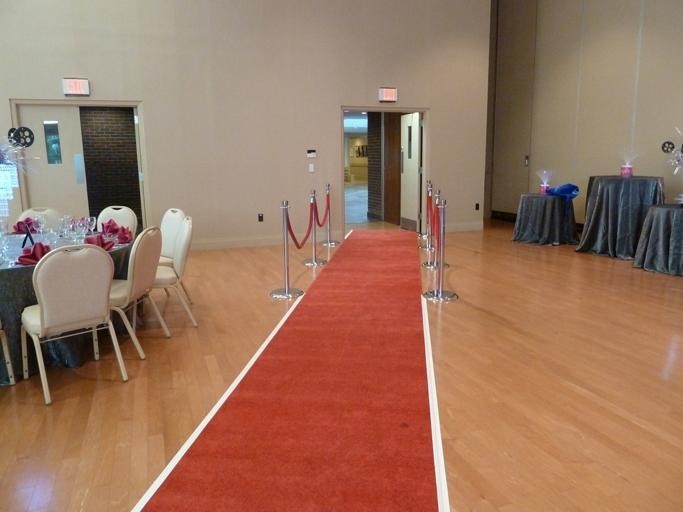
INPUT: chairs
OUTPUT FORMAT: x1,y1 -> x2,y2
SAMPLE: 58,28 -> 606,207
1,203 -> 196,405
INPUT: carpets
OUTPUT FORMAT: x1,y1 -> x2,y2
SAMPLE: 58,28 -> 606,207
130,228 -> 453,512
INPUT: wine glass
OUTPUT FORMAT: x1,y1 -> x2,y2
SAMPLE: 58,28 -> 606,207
31,214 -> 97,244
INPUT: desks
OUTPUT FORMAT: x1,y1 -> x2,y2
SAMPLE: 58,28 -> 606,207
516,175 -> 682,275
